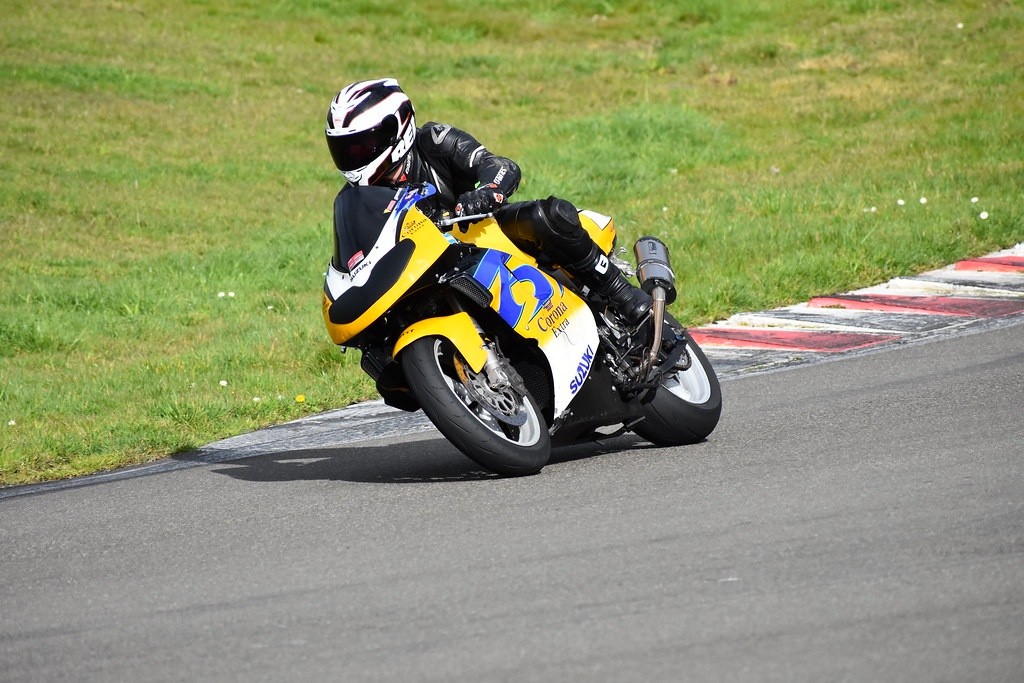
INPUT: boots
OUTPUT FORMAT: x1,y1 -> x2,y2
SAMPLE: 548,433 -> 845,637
560,241 -> 655,323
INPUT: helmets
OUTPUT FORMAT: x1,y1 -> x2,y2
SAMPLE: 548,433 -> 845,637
325,78 -> 417,187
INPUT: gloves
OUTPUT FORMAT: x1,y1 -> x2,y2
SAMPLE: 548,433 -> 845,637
455,183 -> 508,233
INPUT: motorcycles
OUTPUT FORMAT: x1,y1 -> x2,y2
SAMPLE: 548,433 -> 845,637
321,179 -> 722,478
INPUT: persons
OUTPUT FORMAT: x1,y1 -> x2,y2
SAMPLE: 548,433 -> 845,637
326,77 -> 655,413
